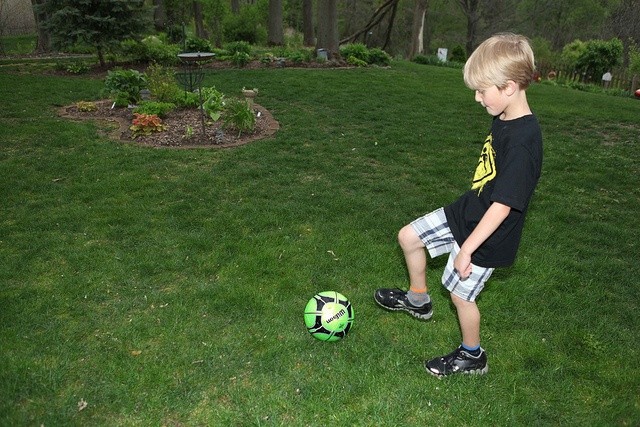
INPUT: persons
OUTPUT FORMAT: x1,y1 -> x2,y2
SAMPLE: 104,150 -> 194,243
374,31 -> 544,379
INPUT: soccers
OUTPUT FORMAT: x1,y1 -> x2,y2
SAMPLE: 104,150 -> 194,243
304,290 -> 354,342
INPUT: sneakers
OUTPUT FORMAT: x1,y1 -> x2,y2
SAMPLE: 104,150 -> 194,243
373,288 -> 434,321
423,345 -> 488,378
